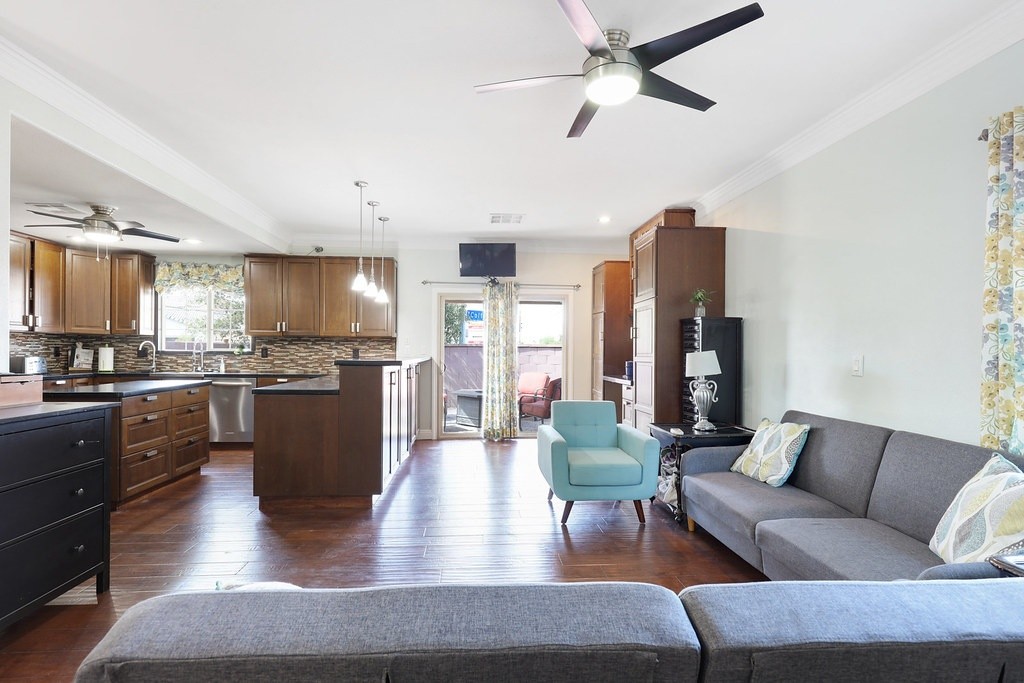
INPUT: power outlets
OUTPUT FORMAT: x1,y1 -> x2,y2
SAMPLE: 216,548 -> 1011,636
53,347 -> 60,358
352,348 -> 359,359
261,347 -> 268,358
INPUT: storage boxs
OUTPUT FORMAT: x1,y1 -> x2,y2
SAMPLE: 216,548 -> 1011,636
0,376 -> 43,405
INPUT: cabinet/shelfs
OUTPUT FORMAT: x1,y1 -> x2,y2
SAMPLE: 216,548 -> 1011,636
252,376 -> 340,499
1,380 -> 213,631
9,230 -> 157,338
591,207 -> 742,434
650,424 -> 755,524
243,254 -> 398,339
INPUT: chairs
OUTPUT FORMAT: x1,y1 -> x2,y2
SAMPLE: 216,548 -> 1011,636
537,401 -> 661,525
519,371 -> 562,431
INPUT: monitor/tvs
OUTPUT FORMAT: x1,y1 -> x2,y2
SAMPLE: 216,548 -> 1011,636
460,243 -> 517,276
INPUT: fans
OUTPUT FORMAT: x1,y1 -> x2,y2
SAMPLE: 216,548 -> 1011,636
471,0 -> 765,137
24,205 -> 180,244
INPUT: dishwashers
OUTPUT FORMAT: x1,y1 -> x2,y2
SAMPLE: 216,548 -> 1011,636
204,377 -> 256,442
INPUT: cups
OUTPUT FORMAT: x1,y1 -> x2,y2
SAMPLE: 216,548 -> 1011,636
625,360 -> 633,380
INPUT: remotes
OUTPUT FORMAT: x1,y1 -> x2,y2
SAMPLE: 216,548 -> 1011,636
670,428 -> 684,436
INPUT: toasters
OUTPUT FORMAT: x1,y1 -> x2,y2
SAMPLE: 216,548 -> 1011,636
10,355 -> 48,375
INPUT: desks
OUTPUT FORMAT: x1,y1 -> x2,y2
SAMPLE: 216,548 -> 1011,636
452,389 -> 482,428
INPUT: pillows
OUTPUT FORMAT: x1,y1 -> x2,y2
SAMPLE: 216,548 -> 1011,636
929,452 -> 1024,566
731,416 -> 811,488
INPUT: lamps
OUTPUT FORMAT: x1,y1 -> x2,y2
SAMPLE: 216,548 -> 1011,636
685,350 -> 723,432
583,63 -> 642,105
351,181 -> 389,304
82,227 -> 121,263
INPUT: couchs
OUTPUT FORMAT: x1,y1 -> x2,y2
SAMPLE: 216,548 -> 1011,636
75,410 -> 1024,683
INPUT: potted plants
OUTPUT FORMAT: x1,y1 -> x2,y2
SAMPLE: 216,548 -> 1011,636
690,288 -> 715,317
